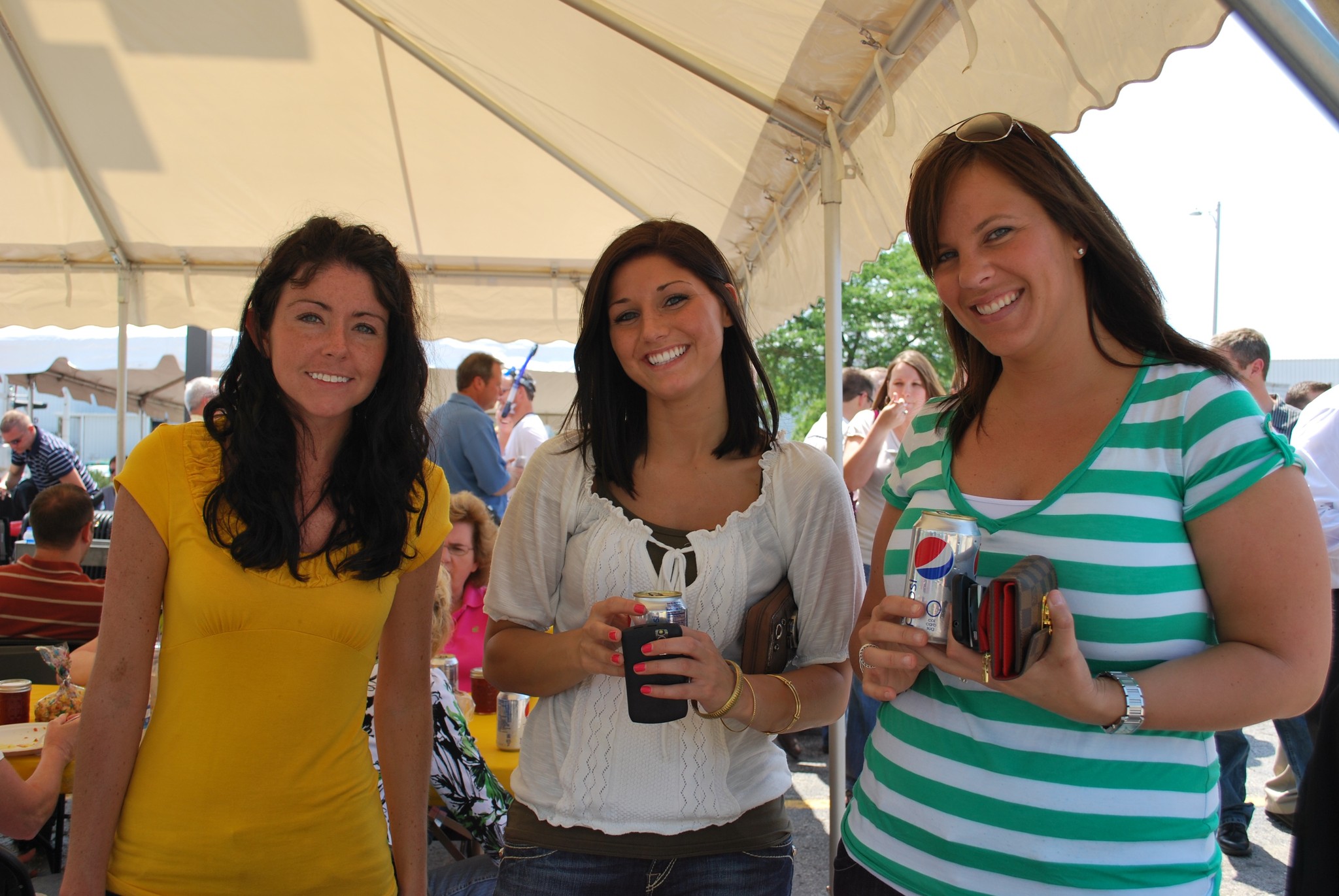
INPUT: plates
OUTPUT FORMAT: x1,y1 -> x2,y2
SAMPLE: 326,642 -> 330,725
0,722 -> 49,757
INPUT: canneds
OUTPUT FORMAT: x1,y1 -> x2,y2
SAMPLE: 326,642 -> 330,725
430,653 -> 458,691
899,511 -> 983,643
495,691 -> 531,751
627,590 -> 688,631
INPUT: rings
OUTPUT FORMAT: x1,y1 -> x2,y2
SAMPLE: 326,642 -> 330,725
959,678 -> 968,683
904,410 -> 908,414
859,644 -> 879,672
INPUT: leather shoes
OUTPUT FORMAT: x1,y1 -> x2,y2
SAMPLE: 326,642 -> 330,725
1218,823 -> 1251,857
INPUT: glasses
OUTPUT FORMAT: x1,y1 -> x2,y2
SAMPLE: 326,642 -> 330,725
3,425 -> 29,444
910,112 -> 1038,183
444,543 -> 478,556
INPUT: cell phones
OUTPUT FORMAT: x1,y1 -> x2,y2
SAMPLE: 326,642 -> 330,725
619,622 -> 688,724
951,573 -> 988,652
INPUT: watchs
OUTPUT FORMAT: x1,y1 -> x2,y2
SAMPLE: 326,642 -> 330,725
1095,671 -> 1145,736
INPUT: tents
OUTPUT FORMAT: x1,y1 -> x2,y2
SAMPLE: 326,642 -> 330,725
0,0 -> 1230,853
1,327 -> 239,429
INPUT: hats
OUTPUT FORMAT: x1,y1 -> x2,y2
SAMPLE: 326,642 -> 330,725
501,367 -> 536,396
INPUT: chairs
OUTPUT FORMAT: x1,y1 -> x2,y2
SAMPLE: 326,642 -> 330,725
0,636 -> 91,864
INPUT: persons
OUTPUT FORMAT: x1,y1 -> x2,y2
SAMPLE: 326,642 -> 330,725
426,352 -> 524,525
59,216 -> 454,896
1206,328 -> 1339,896
439,493 -> 499,692
494,369 -> 549,501
361,564 -> 514,896
0,410 -> 122,896
480,222 -> 865,896
184,376 -> 223,422
835,111 -> 1332,896
803,350 -> 968,799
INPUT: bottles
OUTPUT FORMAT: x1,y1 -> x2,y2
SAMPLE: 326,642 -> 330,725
23,527 -> 35,542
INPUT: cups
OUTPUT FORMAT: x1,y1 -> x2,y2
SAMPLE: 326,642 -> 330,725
470,667 -> 499,715
0,679 -> 32,726
150,642 -> 161,720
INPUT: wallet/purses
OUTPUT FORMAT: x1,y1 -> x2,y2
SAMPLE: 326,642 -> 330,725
978,553 -> 1059,681
740,578 -> 799,683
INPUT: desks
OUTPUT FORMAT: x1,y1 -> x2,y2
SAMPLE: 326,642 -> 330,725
0,683 -> 90,787
457,689 -> 536,802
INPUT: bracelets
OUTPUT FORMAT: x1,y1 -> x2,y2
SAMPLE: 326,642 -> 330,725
691,658 -> 743,720
760,674 -> 801,736
721,677 -> 756,732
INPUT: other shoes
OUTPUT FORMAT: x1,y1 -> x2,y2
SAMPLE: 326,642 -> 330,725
778,731 -> 805,759
1269,800 -> 1300,834
